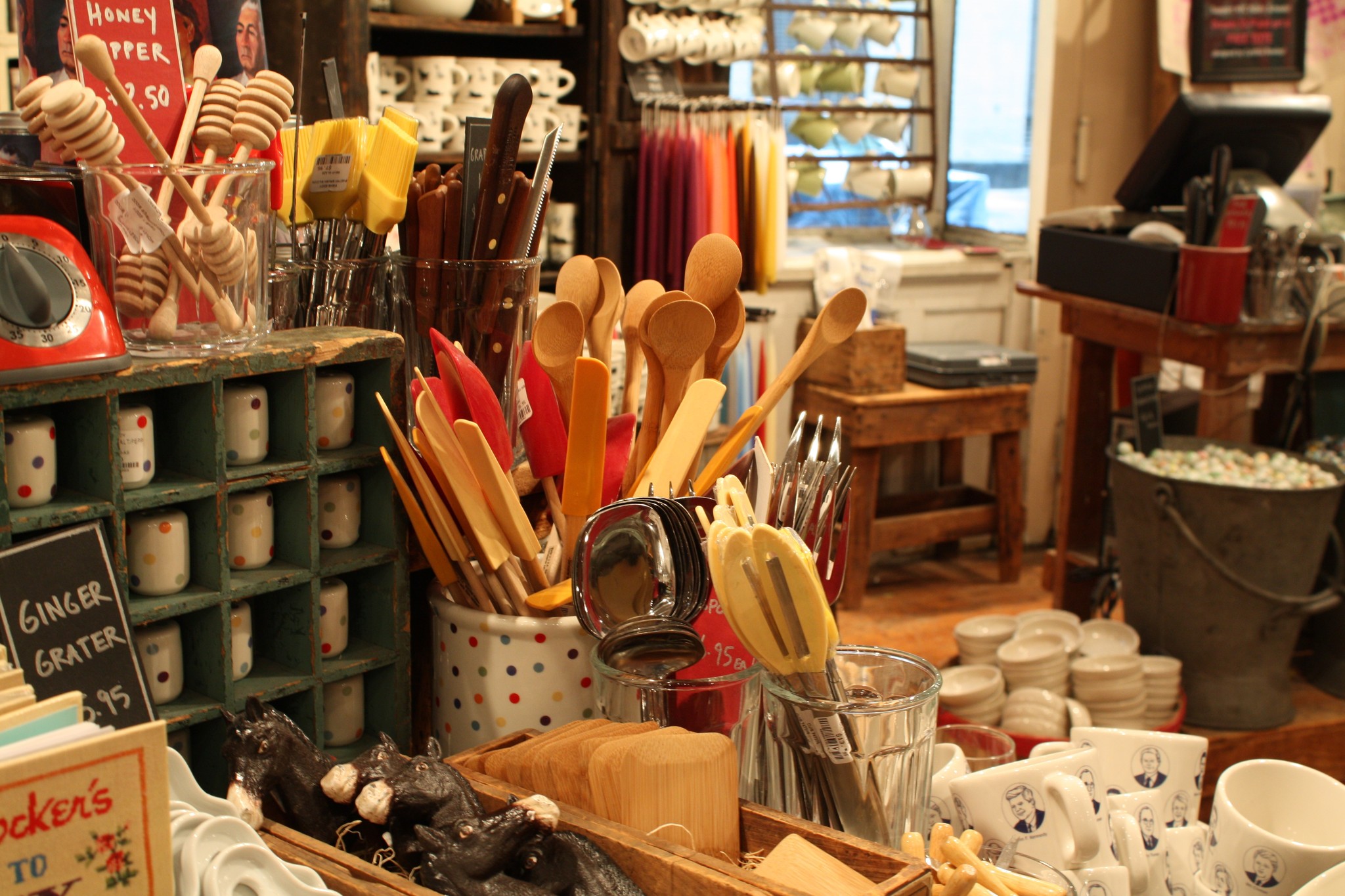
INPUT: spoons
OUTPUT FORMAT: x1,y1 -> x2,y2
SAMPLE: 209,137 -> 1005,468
598,615 -> 705,726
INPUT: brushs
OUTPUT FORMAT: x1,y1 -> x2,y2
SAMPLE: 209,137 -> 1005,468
279,105 -> 426,332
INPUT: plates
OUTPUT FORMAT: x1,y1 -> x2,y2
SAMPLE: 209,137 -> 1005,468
937,609 -> 1182,737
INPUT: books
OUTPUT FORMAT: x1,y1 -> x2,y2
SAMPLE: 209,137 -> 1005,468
0,645 -> 176,896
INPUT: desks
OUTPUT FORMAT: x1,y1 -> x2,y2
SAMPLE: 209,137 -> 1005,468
795,379 -> 1030,608
1016,280 -> 1345,622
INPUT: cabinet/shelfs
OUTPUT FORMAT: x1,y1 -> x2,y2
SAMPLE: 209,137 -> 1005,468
0,0 -> 1031,792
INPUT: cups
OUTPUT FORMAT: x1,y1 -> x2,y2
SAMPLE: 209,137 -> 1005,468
391,250 -> 539,469
762,645 -> 942,851
918,718 -> 1345,896
752,0 -> 933,201
266,243 -> 392,333
617,0 -> 765,68
76,159 -> 276,356
589,639 -> 763,804
425,555 -> 640,759
538,202 -> 574,262
365,52 -> 586,154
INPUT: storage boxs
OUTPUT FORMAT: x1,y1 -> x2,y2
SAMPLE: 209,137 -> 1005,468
798,318 -> 907,393
251,729 -> 936,896
911,344 -> 1037,387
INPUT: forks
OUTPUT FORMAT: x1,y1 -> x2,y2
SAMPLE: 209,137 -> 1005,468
767,410 -> 856,604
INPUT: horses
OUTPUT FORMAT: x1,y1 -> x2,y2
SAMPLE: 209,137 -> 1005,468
218,695 -> 644,896
